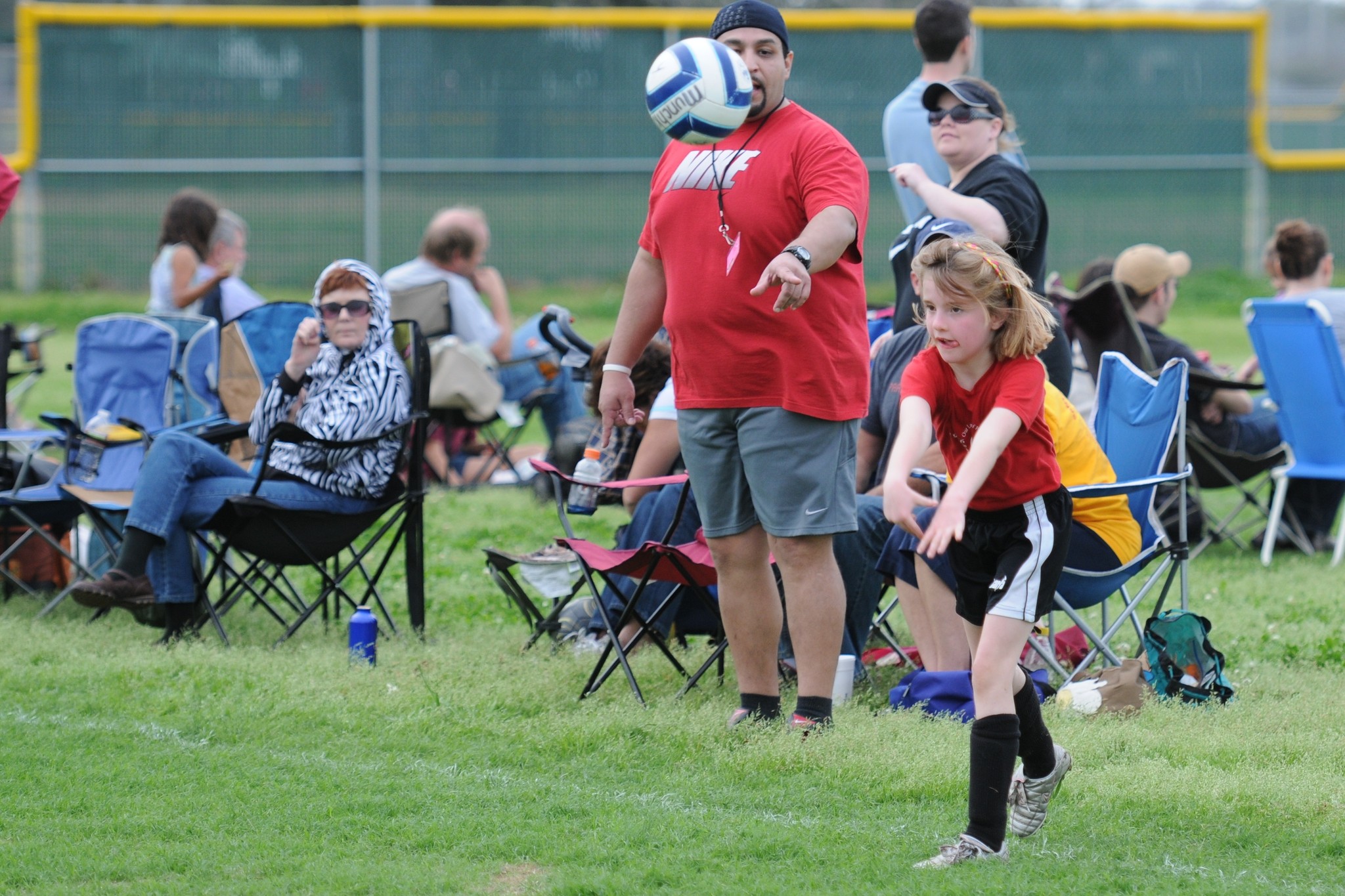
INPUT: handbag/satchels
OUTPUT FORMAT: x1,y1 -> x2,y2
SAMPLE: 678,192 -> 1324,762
1143,609 -> 1236,706
889,663 -> 1047,723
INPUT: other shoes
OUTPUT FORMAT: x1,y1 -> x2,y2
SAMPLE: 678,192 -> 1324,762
69,569 -> 155,609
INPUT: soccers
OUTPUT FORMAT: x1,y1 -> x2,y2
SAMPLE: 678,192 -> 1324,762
644,37 -> 754,146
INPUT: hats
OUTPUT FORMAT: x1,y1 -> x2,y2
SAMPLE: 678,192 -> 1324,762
921,81 -> 1004,120
914,218 -> 973,255
1112,243 -> 1191,298
709,0 -> 789,53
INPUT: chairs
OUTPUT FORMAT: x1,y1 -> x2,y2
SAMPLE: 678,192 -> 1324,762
0,279 -> 730,710
911,265 -> 1345,697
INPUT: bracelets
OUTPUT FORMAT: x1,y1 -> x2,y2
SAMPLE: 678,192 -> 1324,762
602,364 -> 631,375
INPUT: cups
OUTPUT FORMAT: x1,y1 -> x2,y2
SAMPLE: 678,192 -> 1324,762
832,655 -> 855,706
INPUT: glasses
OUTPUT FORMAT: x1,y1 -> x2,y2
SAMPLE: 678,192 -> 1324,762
318,300 -> 371,318
928,104 -> 997,126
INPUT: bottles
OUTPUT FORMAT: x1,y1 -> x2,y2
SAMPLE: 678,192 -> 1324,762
526,337 -> 560,380
566,447 -> 602,514
348,605 -> 378,667
1022,626 -> 1055,682
73,408 -> 112,483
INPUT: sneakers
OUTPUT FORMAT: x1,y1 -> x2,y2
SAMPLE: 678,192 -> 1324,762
1007,744 -> 1071,838
911,840 -> 1009,870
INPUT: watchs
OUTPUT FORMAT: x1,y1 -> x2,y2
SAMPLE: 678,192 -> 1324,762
782,246 -> 811,271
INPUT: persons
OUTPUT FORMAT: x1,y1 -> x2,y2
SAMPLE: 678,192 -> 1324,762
597,0 -> 872,736
380,205 -> 592,487
70,257 -> 412,630
883,234 -> 1071,869
584,331 -> 725,652
202,209 -> 263,325
871,380 -> 1144,673
881,0 -> 1027,227
1269,220 -> 1345,551
775,218 -> 1073,685
889,77 -> 1049,337
1073,242 -> 1284,486
148,190 -> 236,316
